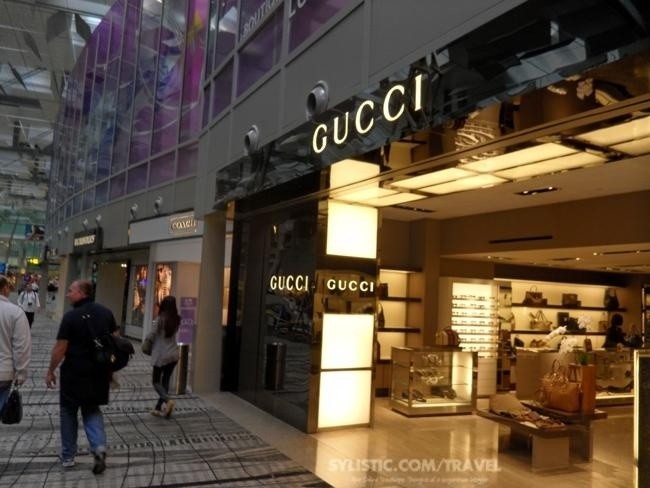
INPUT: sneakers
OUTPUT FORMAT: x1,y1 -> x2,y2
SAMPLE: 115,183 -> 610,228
58,454 -> 76,467
92,452 -> 106,475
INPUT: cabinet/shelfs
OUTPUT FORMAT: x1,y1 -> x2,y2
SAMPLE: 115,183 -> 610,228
314,266 -> 649,416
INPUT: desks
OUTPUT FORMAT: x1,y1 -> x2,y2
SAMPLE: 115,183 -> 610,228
476,409 -> 585,470
521,401 -> 607,463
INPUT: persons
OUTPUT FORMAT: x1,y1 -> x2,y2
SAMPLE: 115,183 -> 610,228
0,275 -> 32,424
142,296 -> 181,420
17,283 -> 40,330
601,314 -> 629,348
46,281 -> 55,304
46,280 -> 119,474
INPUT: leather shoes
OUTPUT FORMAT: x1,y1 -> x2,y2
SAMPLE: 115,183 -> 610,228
150,410 -> 160,417
166,401 -> 176,419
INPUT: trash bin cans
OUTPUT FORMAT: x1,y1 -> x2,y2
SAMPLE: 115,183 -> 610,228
263,342 -> 286,392
166,343 -> 189,396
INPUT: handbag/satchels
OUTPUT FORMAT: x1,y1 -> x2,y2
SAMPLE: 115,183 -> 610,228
378,303 -> 385,329
529,340 -> 544,347
415,369 -> 439,385
543,366 -> 581,413
559,312 -> 587,333
403,389 -> 426,402
431,385 -> 457,399
604,287 -> 620,310
562,293 -> 577,306
446,325 -> 460,346
423,353 -> 442,368
490,393 -> 532,422
531,310 -> 553,331
524,284 -> 547,307
2,380 -> 22,424
598,313 -> 610,333
88,330 -> 134,372
626,325 -> 643,348
436,327 -> 448,347
533,360 -> 561,406
140,338 -> 152,355
